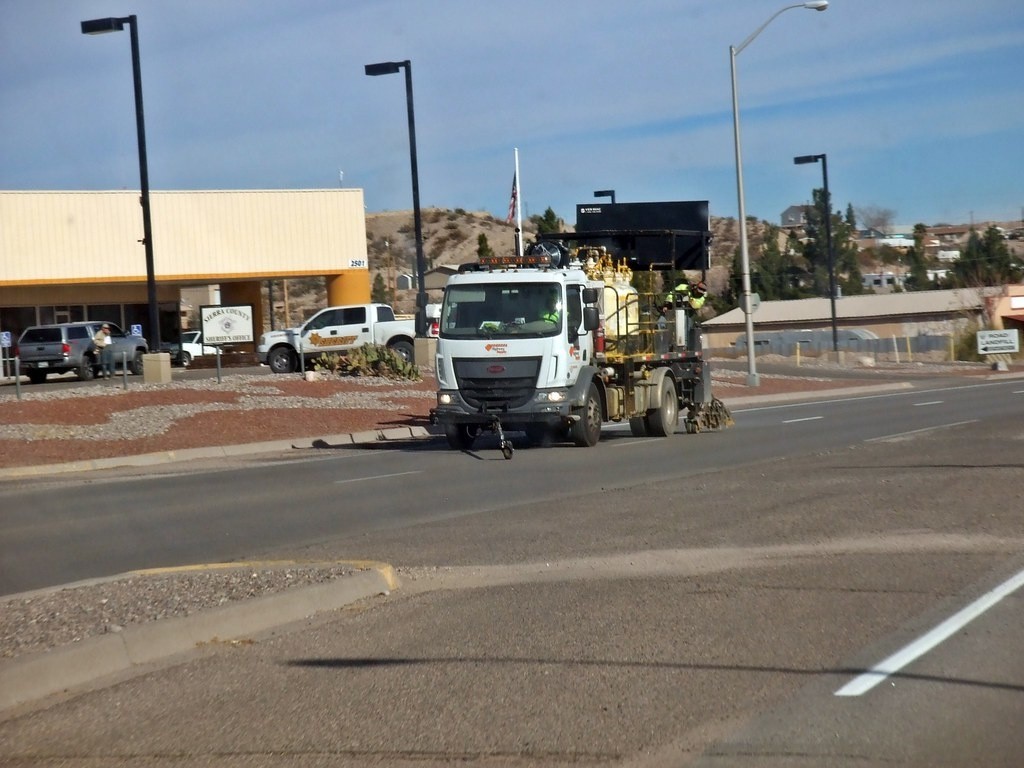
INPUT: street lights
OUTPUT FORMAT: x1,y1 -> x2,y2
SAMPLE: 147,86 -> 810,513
81,14 -> 160,356
794,152 -> 838,351
364,59 -> 430,336
729,2 -> 831,387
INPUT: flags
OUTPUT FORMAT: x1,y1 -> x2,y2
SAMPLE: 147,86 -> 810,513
506,172 -> 517,224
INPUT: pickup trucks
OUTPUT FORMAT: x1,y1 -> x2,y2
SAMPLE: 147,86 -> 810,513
257,302 -> 439,373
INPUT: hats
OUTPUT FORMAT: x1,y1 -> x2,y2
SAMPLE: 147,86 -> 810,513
102,323 -> 109,328
696,281 -> 707,295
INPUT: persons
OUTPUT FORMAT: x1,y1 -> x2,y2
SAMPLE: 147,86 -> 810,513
537,291 -> 570,324
94,324 -> 119,380
658,282 -> 707,331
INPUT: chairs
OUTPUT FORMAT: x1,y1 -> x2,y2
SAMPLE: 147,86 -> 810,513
475,303 -> 499,327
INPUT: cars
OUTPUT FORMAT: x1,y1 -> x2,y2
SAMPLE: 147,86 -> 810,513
161,331 -> 222,368
15,320 -> 148,383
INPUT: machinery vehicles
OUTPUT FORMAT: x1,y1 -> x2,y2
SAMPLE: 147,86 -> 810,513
429,236 -> 713,459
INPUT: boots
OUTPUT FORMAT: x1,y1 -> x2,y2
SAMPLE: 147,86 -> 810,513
103,371 -> 118,381
